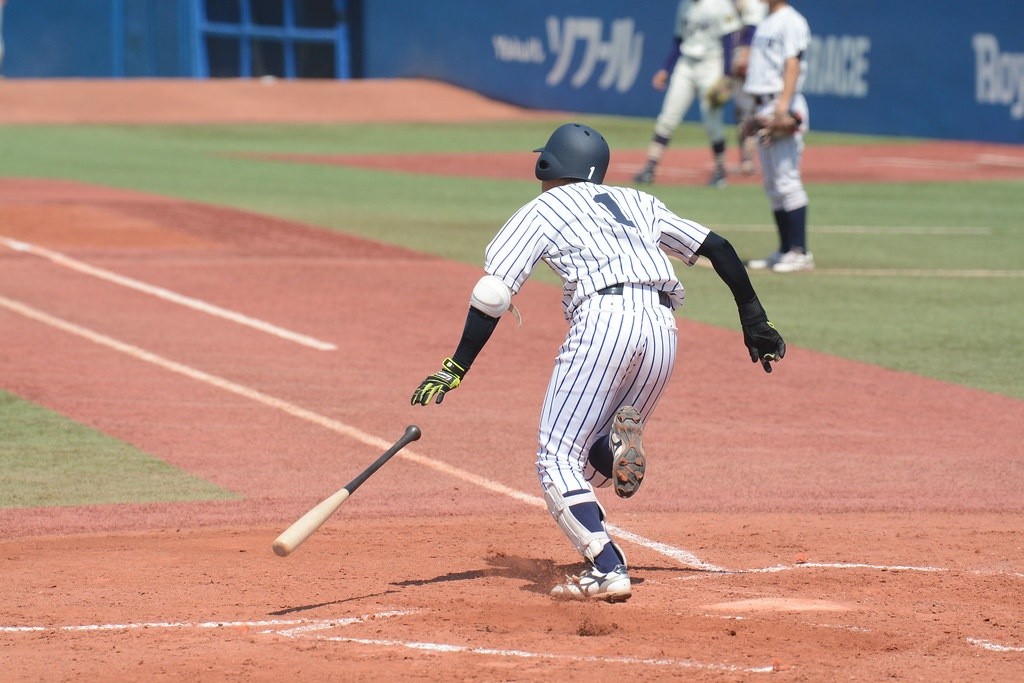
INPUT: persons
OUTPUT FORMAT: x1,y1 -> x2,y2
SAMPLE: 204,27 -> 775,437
409,122 -> 788,604
630,0 -> 818,274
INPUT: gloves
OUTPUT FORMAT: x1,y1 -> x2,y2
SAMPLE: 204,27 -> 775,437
743,321 -> 786,374
411,357 -> 466,406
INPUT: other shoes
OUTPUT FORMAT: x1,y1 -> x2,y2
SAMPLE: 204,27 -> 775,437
747,248 -> 783,271
708,168 -> 726,189
635,168 -> 653,184
772,251 -> 815,274
741,159 -> 756,176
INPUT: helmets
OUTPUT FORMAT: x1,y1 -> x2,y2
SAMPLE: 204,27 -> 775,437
532,122 -> 610,184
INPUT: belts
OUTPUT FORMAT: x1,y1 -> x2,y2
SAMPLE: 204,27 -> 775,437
754,94 -> 774,105
597,282 -> 676,312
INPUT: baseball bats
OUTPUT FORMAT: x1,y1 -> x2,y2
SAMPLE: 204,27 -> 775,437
271,424 -> 422,558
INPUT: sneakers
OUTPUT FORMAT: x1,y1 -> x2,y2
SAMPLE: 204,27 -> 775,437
607,405 -> 646,498
550,564 -> 633,602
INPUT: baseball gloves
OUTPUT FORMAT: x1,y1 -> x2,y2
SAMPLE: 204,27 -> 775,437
736,110 -> 804,149
